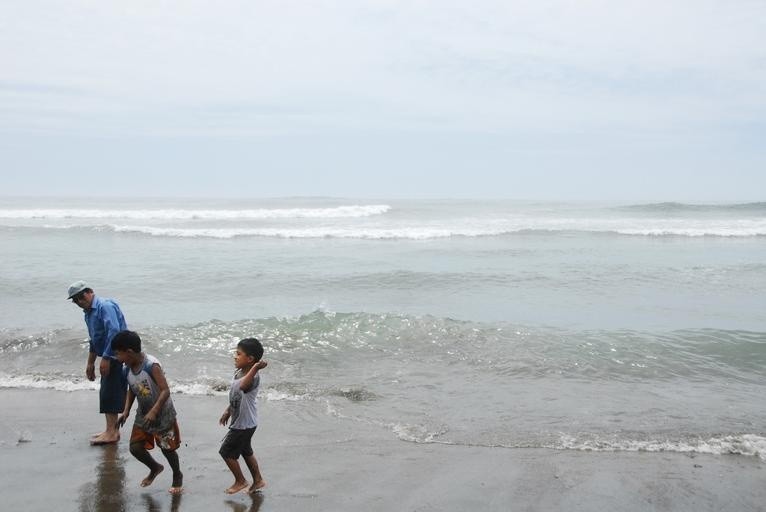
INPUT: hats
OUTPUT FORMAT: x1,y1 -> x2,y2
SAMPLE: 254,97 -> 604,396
67,281 -> 92,300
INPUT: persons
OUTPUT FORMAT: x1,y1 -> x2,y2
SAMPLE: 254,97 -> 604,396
66,279 -> 127,444
217,337 -> 268,494
111,329 -> 184,494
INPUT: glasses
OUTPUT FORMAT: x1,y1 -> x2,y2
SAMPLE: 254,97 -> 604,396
72,291 -> 89,303
